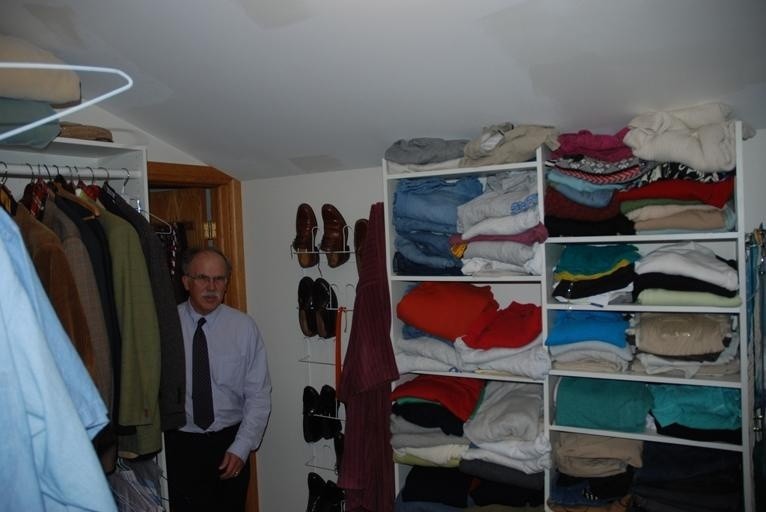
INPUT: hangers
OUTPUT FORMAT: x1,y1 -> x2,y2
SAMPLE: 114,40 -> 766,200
53,162 -> 101,221
26,161 -> 34,184
42,163 -> 50,181
64,164 -> 75,187
0,162 -> 59,243
97,164 -> 110,187
74,165 -> 82,186
0,60 -> 133,141
118,167 -> 171,236
34,162 -> 42,182
85,166 -> 95,187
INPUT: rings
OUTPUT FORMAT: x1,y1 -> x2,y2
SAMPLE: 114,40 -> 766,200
234,470 -> 240,475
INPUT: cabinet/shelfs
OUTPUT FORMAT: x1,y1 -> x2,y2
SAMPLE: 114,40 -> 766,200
287,222 -> 365,507
1,129 -> 172,512
379,123 -> 756,511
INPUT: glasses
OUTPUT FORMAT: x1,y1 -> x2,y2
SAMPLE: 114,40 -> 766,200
190,273 -> 228,287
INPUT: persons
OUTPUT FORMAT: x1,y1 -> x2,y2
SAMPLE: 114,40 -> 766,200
166,247 -> 270,512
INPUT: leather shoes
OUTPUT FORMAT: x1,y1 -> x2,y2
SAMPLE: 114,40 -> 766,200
313,278 -> 338,339
320,203 -> 350,268
301,385 -> 322,444
292,203 -> 320,268
297,276 -> 317,337
305,471 -> 342,512
317,384 -> 342,440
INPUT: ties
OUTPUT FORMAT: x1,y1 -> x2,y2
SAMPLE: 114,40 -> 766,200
192,317 -> 215,431
